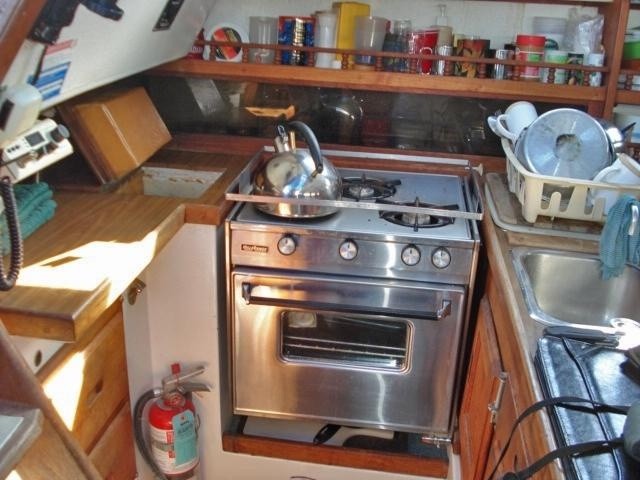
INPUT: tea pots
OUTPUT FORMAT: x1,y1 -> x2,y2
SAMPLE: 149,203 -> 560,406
251,120 -> 344,220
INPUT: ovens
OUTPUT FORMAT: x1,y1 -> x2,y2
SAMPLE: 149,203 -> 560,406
230,266 -> 467,437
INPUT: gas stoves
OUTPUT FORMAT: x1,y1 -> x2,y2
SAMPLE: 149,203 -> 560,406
229,166 -> 475,288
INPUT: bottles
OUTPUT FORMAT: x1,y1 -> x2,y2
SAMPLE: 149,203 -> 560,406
542,33 -> 606,86
393,19 -> 414,73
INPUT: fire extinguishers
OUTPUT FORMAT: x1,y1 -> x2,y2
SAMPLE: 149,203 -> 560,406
134,363 -> 211,480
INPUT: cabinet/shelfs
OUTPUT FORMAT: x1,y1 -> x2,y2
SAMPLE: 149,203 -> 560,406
453,270 -> 535,479
21,294 -> 140,480
146,0 -> 640,160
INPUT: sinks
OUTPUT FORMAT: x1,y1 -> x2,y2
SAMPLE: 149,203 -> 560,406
105,167 -> 225,200
516,247 -> 640,329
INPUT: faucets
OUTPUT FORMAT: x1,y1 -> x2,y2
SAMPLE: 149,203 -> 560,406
626,203 -> 639,236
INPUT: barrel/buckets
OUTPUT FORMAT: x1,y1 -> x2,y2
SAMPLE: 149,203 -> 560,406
510,108 -> 616,208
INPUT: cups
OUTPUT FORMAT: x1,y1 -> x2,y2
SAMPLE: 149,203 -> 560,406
495,101 -> 539,148
351,14 -> 389,66
246,15 -> 279,65
588,152 -> 640,217
